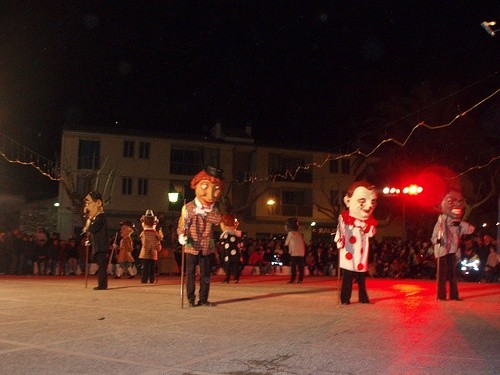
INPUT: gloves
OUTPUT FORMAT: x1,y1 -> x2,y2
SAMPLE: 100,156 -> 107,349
452,221 -> 460,226
193,207 -> 207,219
437,238 -> 441,244
84,240 -> 91,246
178,233 -> 189,246
337,241 -> 343,249
354,219 -> 366,227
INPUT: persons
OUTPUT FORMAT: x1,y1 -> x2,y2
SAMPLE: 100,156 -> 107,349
177,166 -> 221,306
0,209 -> 500,285
334,181 -> 377,305
431,193 -> 474,302
83,191 -> 109,290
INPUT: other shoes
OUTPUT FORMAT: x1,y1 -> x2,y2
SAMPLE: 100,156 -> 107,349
297,280 -> 302,285
222,277 -> 230,284
451,297 -> 461,301
288,279 -> 295,284
188,301 -> 194,306
359,298 -> 370,304
128,275 -> 135,279
112,275 -> 121,279
342,300 -> 350,305
93,286 -> 106,290
199,300 -> 216,307
233,278 -> 238,284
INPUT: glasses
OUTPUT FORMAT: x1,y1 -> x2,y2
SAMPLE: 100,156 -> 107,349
85,201 -> 94,206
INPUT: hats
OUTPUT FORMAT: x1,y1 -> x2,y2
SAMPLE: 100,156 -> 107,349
120,220 -> 138,232
